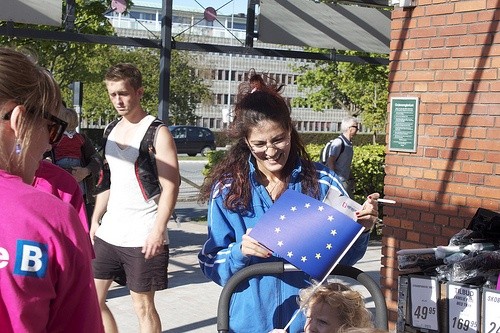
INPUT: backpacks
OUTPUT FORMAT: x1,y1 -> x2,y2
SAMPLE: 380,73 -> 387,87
320,136 -> 345,166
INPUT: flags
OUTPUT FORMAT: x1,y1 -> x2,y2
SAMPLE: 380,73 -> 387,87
247,188 -> 364,281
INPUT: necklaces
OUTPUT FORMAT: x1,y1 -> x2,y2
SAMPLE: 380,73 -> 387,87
256,169 -> 281,195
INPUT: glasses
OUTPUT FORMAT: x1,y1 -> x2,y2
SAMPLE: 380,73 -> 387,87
2,104 -> 68,145
351,126 -> 358,129
247,128 -> 291,153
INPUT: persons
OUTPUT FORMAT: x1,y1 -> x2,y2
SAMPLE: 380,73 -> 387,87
0,47 -> 103,333
90,64 -> 181,333
268,282 -> 374,333
323,115 -> 358,200
196,75 -> 380,333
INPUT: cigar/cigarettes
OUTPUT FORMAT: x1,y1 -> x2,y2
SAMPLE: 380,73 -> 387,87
374,198 -> 396,204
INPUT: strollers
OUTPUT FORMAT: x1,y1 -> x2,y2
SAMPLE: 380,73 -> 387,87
215,260 -> 389,333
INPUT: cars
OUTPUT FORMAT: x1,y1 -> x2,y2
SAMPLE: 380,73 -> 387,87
167,125 -> 217,157
